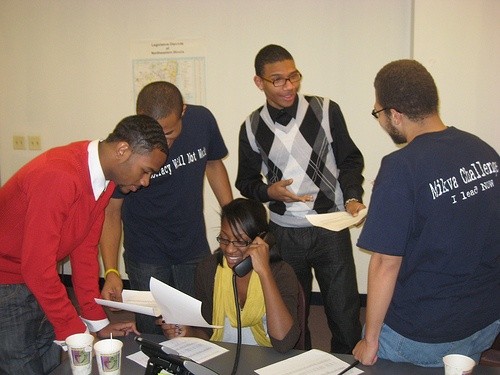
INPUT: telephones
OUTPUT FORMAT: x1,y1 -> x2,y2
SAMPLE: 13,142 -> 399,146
232,231 -> 276,278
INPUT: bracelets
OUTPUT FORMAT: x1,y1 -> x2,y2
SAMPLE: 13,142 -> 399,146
104,269 -> 120,279
345,198 -> 358,206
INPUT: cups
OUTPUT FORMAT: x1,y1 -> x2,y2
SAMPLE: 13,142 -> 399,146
442,354 -> 476,375
93,338 -> 123,375
63,333 -> 95,375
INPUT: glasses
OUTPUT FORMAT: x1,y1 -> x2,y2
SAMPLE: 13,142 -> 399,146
371,107 -> 403,118
216,234 -> 251,247
257,69 -> 302,86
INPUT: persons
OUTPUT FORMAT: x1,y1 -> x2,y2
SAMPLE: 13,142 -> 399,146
156,198 -> 305,353
0,114 -> 169,375
352,59 -> 500,368
97,81 -> 234,334
235,44 -> 364,355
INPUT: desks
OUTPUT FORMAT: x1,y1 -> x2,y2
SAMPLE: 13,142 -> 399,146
45,332 -> 358,375
343,358 -> 500,375
